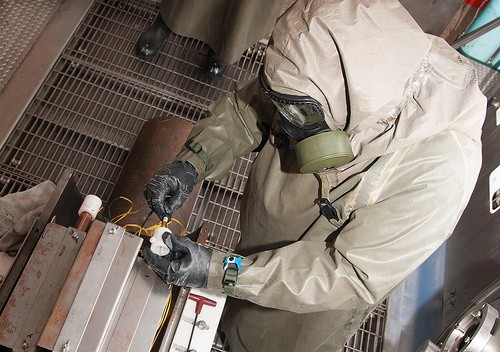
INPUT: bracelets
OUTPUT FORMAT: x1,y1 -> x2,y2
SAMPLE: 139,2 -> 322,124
222,255 -> 243,298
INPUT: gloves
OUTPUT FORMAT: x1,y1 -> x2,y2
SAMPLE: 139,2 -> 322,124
143,161 -> 198,222
139,232 -> 213,289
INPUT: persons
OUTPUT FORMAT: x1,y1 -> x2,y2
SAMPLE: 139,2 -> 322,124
141,0 -> 487,352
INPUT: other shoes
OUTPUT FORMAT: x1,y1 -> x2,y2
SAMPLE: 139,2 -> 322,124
205,47 -> 228,78
135,12 -> 172,58
